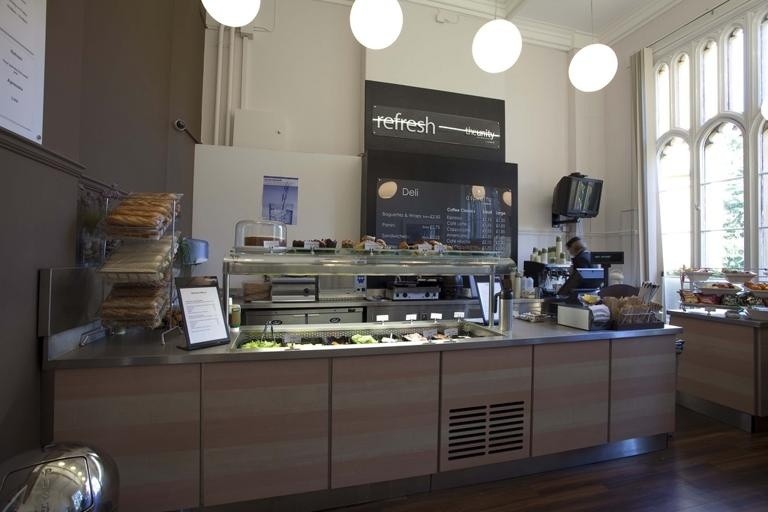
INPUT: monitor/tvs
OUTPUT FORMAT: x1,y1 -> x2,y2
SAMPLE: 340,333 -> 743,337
523,261 -> 548,288
551,176 -> 603,218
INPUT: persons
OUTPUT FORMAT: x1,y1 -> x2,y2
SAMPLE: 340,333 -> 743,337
566,236 -> 602,268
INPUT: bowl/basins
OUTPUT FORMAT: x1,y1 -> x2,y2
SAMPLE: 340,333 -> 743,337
685,270 -> 715,281
723,271 -> 757,283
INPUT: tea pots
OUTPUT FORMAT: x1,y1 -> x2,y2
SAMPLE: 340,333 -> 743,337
493,289 -> 514,330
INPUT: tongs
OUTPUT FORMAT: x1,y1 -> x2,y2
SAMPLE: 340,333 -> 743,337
258,320 -> 276,346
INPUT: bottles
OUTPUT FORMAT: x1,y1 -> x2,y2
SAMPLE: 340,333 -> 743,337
522,274 -> 534,292
544,268 -> 569,285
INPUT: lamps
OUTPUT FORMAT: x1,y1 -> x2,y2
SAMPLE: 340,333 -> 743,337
200,0 -> 260,28
472,0 -> 523,73
567,0 -> 618,93
349,0 -> 404,50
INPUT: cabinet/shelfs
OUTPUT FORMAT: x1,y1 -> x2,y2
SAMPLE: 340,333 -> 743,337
222,247 -> 518,333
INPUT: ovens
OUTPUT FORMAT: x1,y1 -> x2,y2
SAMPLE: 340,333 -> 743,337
244,308 -> 365,325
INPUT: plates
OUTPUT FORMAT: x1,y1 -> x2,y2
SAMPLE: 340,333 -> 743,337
698,284 -> 742,295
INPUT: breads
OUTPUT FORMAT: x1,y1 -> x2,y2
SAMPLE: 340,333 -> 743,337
98,270 -> 176,328
743,281 -> 768,291
107,192 -> 181,240
99,234 -> 179,285
292,237 -> 337,248
399,239 -> 453,251
341,235 -> 386,249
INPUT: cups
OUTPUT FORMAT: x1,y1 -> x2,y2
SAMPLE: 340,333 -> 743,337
530,234 -> 567,263
510,264 -> 521,299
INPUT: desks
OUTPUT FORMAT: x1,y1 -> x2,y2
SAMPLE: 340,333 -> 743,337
667,309 -> 768,434
